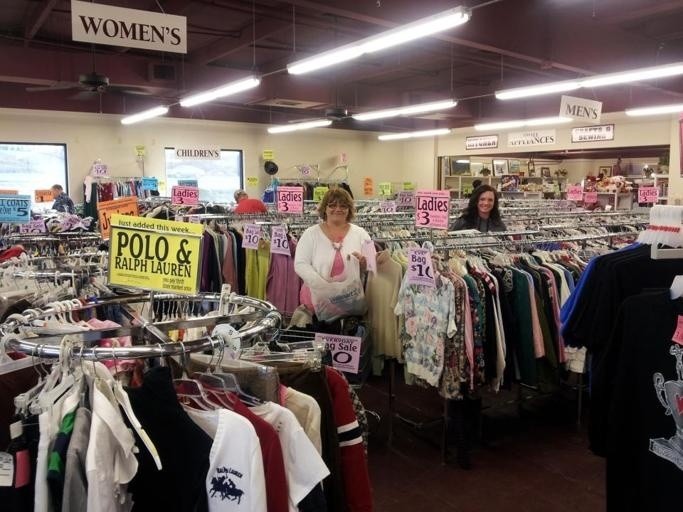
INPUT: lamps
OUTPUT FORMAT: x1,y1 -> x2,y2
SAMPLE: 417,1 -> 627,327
285,1 -> 472,77
623,87 -> 682,117
494,54 -> 681,102
266,86 -> 338,135
178,2 -> 264,110
349,41 -> 459,122
473,97 -> 575,132
120,88 -> 168,128
376,117 -> 453,139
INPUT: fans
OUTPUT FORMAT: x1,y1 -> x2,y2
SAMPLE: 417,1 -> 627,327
26,46 -> 149,96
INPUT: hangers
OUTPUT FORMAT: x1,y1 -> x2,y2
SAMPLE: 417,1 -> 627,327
328,197 -> 660,290
1,196 -> 330,417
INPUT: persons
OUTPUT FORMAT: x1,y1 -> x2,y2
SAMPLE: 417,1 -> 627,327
449,184 -> 508,231
230,187 -> 269,215
292,187 -> 377,330
49,183 -> 75,215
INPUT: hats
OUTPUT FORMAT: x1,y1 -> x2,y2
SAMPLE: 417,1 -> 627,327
262,160 -> 280,176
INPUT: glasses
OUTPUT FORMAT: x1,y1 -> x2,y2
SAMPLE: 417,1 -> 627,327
326,202 -> 350,210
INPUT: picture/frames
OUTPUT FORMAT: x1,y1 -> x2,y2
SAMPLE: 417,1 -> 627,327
492,159 -> 509,176
599,166 -> 612,177
541,167 -> 550,176
